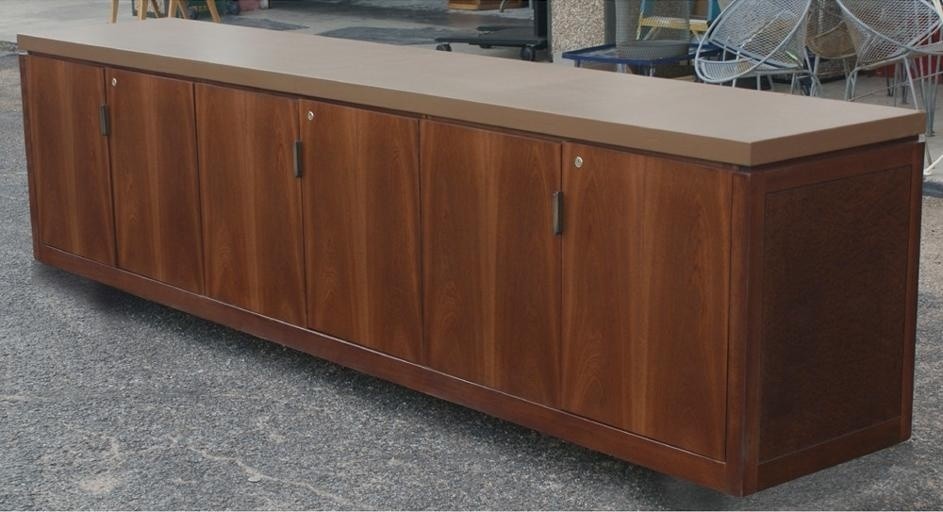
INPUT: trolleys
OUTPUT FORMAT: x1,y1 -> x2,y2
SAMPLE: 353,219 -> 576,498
434,0 -> 548,60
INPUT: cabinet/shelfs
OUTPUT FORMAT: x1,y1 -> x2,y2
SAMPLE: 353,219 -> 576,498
16,18 -> 928,499
